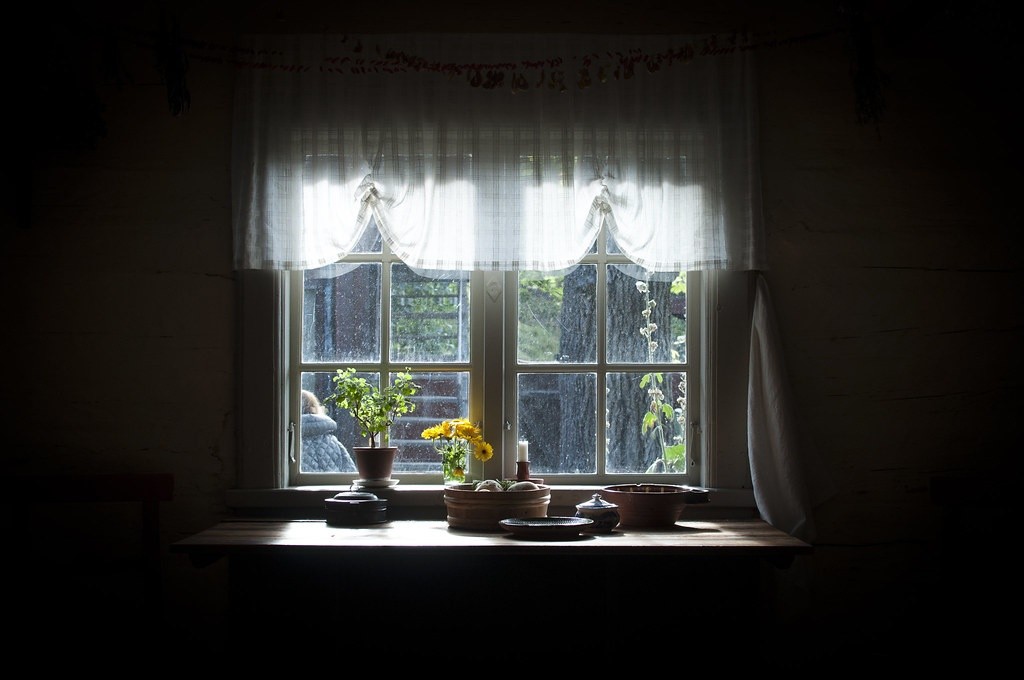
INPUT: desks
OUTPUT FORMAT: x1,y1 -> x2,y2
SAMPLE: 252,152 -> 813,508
171,518 -> 814,546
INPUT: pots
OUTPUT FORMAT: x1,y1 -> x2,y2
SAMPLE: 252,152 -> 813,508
606,483 -> 710,528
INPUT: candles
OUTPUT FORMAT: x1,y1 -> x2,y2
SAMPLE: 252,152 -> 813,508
518,439 -> 528,462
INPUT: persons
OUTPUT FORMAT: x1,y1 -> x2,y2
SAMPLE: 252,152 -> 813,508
301,390 -> 357,472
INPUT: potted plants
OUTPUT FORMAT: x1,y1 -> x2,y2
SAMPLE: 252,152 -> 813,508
321,367 -> 422,481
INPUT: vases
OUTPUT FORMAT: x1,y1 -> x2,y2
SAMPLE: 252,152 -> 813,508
443,463 -> 465,486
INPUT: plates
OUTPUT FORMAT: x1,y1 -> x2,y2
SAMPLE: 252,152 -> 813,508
500,516 -> 594,536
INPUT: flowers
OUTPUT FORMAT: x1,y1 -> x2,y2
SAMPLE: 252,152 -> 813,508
422,417 -> 494,482
